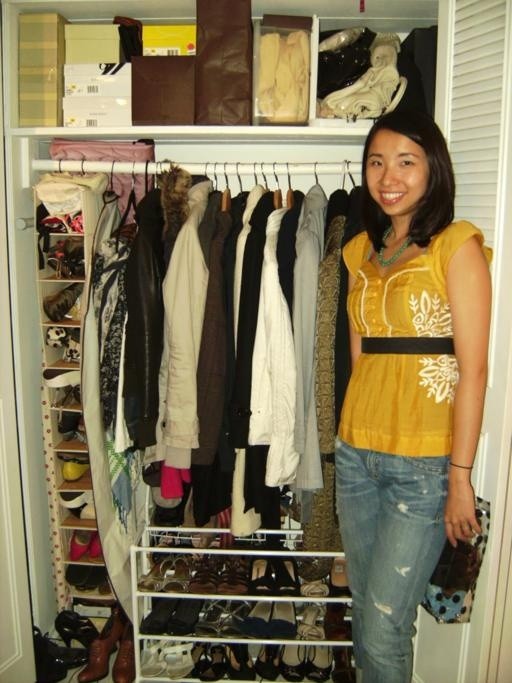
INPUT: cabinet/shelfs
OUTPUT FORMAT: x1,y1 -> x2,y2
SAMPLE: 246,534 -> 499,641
130,527 -> 355,683
10,1 -> 438,133
32,175 -> 121,620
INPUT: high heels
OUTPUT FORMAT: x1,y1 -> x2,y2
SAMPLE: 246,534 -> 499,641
55,610 -> 118,654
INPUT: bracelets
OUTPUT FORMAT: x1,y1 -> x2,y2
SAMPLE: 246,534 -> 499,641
449,462 -> 473,470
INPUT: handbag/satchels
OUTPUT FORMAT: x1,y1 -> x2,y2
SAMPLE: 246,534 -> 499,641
418,496 -> 490,627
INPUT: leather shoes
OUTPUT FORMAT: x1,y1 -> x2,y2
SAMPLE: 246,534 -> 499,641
111,622 -> 135,683
78,614 -> 124,683
34,626 -> 89,667
32,636 -> 67,683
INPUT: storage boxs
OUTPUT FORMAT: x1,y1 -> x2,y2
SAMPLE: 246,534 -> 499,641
55,157 -> 64,176
129,56 -> 200,125
64,63 -> 133,99
61,98 -> 130,128
141,25 -> 197,56
252,19 -> 312,127
64,24 -> 125,63
16,13 -> 72,127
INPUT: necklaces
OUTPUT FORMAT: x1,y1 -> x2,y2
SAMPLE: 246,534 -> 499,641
378,223 -> 415,266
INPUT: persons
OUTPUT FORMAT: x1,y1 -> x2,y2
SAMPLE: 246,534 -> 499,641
335,109 -> 492,683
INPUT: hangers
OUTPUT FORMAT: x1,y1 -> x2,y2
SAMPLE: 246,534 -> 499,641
247,155 -> 259,209
280,160 -> 300,214
80,155 -> 86,175
115,155 -> 138,255
232,159 -> 245,195
260,158 -> 269,191
201,158 -> 218,211
339,152 -> 359,194
270,159 -> 284,213
221,158 -> 232,214
312,161 -> 320,192
108,152 -> 116,203
142,154 -> 176,219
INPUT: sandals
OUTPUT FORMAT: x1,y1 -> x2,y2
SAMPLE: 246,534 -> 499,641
191,642 -> 211,678
330,558 -> 351,597
189,555 -> 220,594
306,645 -> 332,682
161,552 -> 192,593
225,644 -> 256,681
279,645 -> 306,682
194,600 -> 227,636
271,558 -> 299,597
163,641 -> 195,679
141,598 -> 177,635
62,461 -> 89,482
332,646 -> 356,683
268,601 -> 297,639
216,555 -> 248,594
300,578 -> 329,597
140,639 -> 167,677
324,603 -> 352,641
248,557 -> 271,596
89,532 -> 103,558
219,600 -> 253,639
138,552 -> 175,592
68,530 -> 90,561
242,600 -> 274,636
199,645 -> 226,681
167,599 -> 202,636
254,644 -> 280,681
296,602 -> 324,641
